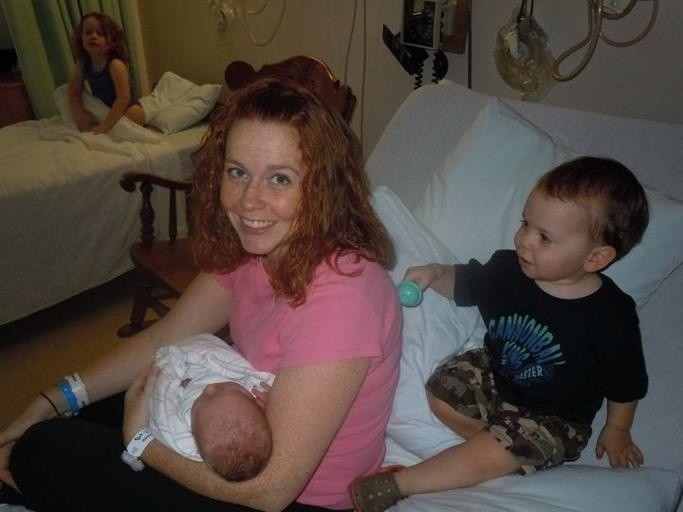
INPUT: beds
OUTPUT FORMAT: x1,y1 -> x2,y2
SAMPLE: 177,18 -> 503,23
0,104 -> 217,326
0,79 -> 683,512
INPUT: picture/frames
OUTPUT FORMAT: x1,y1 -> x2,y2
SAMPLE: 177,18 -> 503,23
399,0 -> 440,50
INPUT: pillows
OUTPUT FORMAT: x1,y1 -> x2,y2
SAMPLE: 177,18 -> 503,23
136,70 -> 224,136
408,98 -> 683,336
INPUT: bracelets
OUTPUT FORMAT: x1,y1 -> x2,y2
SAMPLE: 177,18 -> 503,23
63,372 -> 90,409
39,392 -> 60,417
57,382 -> 79,415
120,428 -> 155,472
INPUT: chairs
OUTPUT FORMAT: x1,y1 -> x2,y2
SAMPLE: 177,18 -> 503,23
116,54 -> 358,338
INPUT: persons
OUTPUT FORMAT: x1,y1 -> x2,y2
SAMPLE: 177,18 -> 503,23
0,75 -> 404,511
65,12 -> 145,134
148,331 -> 276,482
347,156 -> 651,511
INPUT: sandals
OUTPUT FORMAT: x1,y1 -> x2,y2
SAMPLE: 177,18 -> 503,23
349,473 -> 406,511
